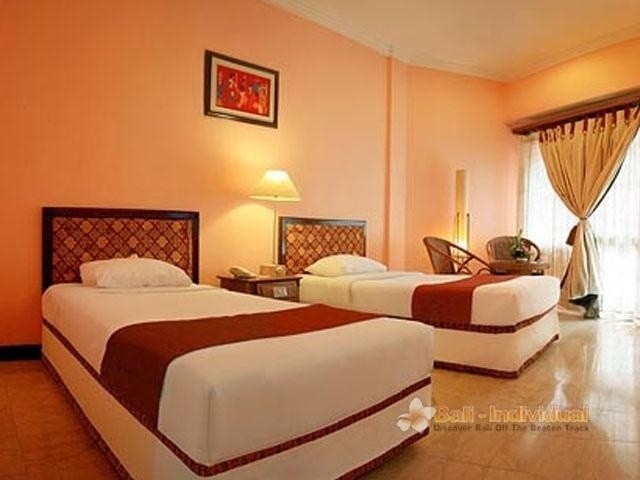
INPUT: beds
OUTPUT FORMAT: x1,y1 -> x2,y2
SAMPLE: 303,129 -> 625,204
41,206 -> 562,480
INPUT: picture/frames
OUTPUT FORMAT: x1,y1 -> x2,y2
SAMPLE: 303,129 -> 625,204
204,48 -> 280,130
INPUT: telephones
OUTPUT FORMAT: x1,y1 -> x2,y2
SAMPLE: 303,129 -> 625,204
229,266 -> 257,278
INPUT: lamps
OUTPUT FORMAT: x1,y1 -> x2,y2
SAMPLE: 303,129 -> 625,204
248,169 -> 302,277
